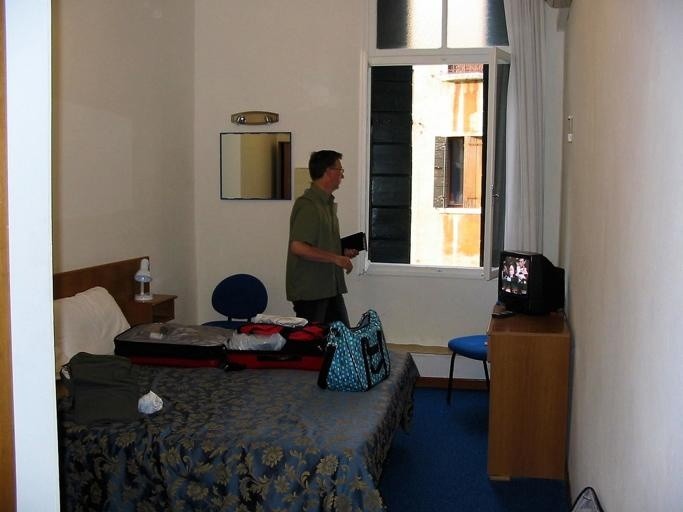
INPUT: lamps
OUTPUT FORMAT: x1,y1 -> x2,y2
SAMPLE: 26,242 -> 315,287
133,257 -> 154,302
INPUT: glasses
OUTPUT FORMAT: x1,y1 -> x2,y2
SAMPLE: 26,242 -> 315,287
331,166 -> 345,175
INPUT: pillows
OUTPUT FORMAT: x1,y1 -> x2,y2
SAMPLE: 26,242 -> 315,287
54,285 -> 131,380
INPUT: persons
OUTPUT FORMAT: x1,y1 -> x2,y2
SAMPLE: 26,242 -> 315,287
284,147 -> 360,330
503,258 -> 528,282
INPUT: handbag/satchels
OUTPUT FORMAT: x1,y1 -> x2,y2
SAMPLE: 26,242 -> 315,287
317,310 -> 391,392
59,351 -> 141,422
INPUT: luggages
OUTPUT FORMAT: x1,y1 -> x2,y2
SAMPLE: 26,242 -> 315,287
114,323 -> 326,371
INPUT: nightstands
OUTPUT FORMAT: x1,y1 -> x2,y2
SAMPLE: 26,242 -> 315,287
129,293 -> 177,325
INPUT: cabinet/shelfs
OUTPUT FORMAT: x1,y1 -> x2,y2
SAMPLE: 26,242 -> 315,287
485,306 -> 570,482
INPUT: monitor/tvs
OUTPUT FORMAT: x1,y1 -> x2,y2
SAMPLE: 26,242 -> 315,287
498,250 -> 566,315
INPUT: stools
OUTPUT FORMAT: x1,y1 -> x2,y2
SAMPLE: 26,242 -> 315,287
447,335 -> 491,407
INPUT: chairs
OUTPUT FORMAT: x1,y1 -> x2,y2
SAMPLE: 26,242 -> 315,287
201,274 -> 267,331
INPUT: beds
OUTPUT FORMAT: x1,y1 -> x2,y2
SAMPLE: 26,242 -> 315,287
53,256 -> 419,512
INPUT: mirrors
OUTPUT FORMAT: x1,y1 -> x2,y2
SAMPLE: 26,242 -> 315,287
218,132 -> 292,201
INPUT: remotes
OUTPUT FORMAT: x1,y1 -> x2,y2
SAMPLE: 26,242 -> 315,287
491,311 -> 514,319
